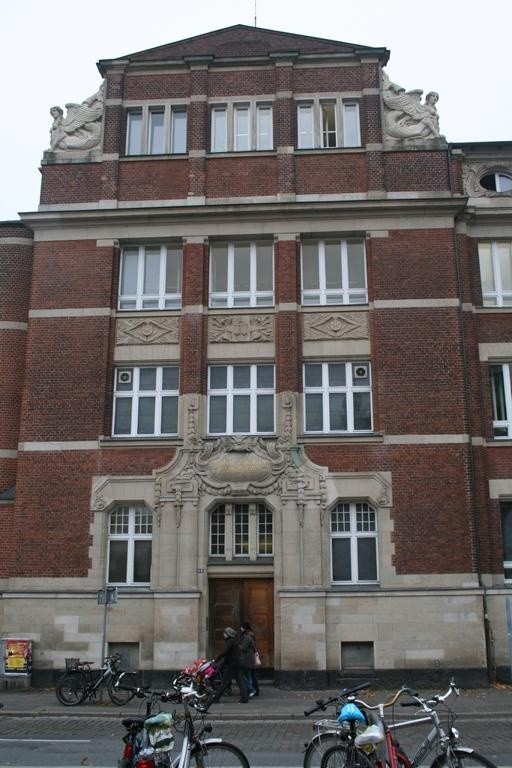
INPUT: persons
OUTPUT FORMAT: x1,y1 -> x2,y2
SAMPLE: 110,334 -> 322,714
412,90 -> 447,140
48,105 -> 68,151
221,620 -> 258,699
248,628 -> 261,696
208,626 -> 250,704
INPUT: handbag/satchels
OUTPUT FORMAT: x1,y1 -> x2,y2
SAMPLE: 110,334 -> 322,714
254,651 -> 262,670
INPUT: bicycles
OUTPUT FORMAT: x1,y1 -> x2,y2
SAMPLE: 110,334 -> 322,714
57,653 -> 138,707
295,677 -> 505,767
117,652 -> 254,767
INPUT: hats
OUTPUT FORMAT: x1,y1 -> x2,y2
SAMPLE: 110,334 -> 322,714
224,627 -> 238,638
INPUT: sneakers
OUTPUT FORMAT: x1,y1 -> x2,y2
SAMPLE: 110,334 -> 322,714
237,689 -> 259,704
